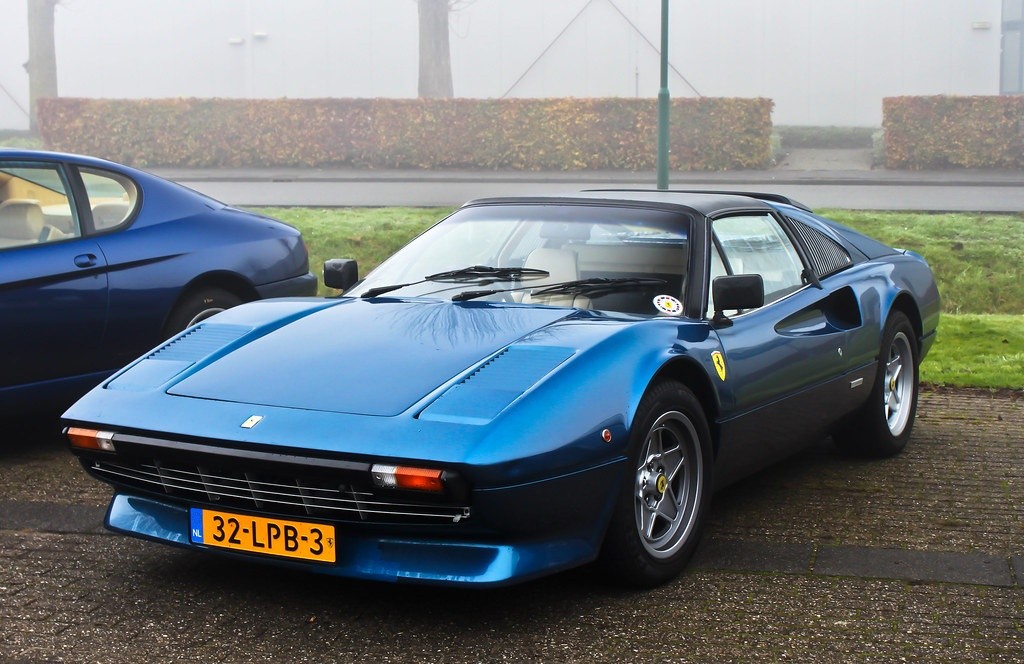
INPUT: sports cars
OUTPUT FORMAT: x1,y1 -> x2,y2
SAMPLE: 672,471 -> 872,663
60,187 -> 941,591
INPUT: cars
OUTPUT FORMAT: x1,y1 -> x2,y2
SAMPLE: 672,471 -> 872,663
0,148 -> 319,434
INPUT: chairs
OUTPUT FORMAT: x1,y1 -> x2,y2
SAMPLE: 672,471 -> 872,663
0,200 -> 778,327
